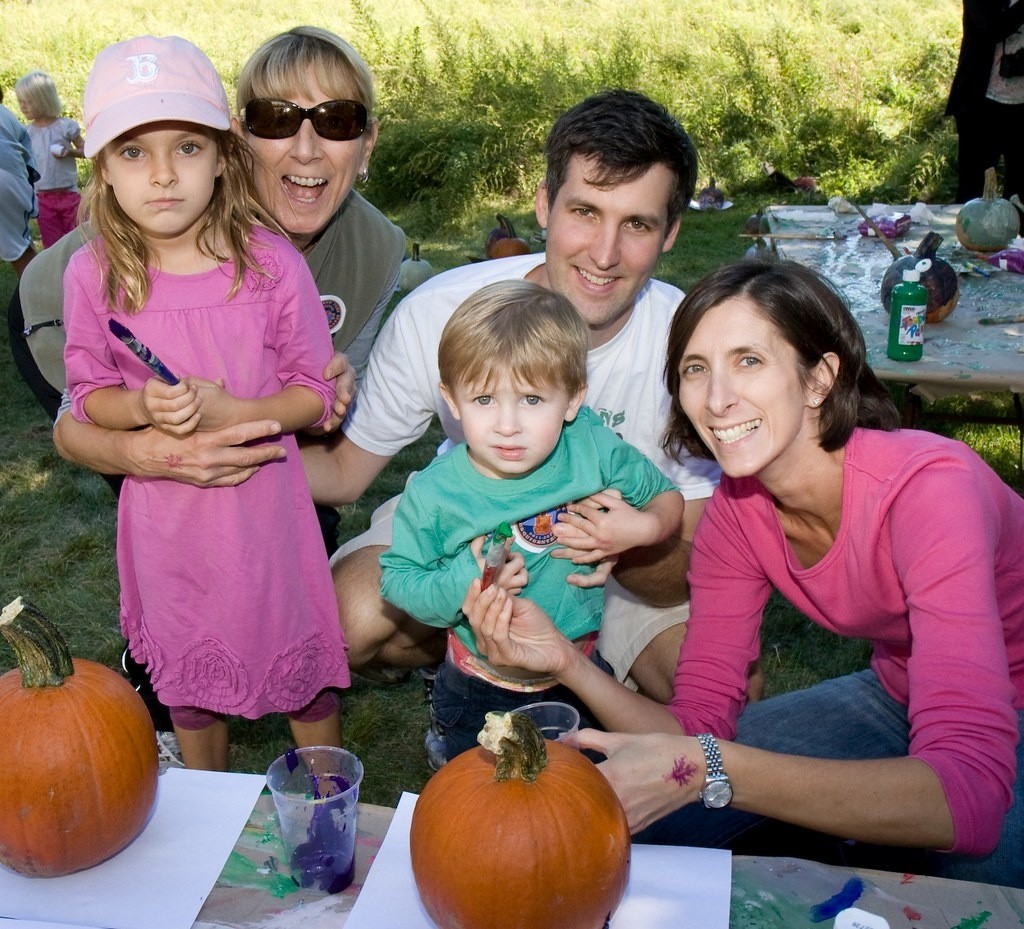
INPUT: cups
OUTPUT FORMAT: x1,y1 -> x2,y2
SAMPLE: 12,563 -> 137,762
266,745 -> 364,895
510,701 -> 581,755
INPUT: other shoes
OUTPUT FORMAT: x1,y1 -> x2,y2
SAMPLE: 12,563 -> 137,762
156,728 -> 187,769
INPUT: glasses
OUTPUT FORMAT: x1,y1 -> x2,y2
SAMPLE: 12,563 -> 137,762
237,96 -> 372,142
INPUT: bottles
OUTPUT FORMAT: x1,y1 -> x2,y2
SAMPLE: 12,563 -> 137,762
887,258 -> 933,362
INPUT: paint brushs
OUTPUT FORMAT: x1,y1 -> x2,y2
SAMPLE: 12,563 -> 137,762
736,231 -> 848,240
953,248 -> 988,259
961,259 -> 990,278
977,314 -> 1024,324
480,521 -> 514,594
107,319 -> 179,386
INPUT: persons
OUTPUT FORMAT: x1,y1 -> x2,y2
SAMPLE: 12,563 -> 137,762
0,71 -> 84,279
378,280 -> 684,764
462,258 -> 1024,889
7,26 -> 411,728
63,35 -> 351,800
299,86 -> 767,771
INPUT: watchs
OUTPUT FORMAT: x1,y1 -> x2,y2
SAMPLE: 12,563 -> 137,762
697,733 -> 732,808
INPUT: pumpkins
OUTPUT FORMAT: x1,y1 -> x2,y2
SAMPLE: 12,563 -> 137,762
400,241 -> 434,291
486,213 -> 529,258
956,167 -> 1020,253
881,231 -> 959,323
409,711 -> 632,929
0,595 -> 159,878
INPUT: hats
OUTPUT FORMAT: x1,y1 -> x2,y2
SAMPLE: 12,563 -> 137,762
83,34 -> 234,159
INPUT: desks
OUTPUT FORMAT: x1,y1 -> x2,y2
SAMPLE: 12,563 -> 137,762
760,203 -> 1024,474
0,793 -> 1024,929
